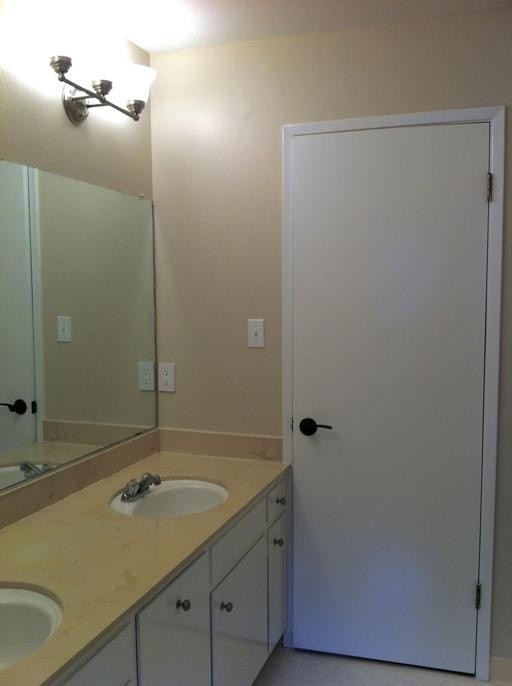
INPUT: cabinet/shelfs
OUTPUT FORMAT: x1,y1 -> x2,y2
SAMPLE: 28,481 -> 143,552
56,477 -> 289,686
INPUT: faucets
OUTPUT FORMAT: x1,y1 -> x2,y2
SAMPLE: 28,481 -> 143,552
139,474 -> 161,492
19,460 -> 40,474
0,462 -> 51,487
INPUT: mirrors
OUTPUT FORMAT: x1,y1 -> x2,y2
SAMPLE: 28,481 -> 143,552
0,159 -> 159,494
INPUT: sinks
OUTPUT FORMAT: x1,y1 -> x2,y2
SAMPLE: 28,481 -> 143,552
110,476 -> 229,517
1,579 -> 63,673
